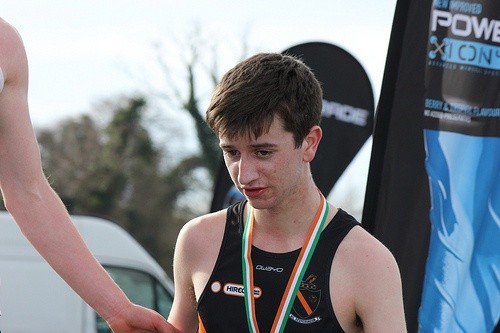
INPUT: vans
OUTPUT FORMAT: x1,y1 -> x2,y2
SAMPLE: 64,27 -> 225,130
0,211 -> 176,333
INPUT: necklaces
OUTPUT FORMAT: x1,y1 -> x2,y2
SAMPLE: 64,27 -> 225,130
242,188 -> 329,333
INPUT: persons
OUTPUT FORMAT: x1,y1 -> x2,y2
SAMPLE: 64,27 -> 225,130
0,16 -> 174,333
166,53 -> 407,333
